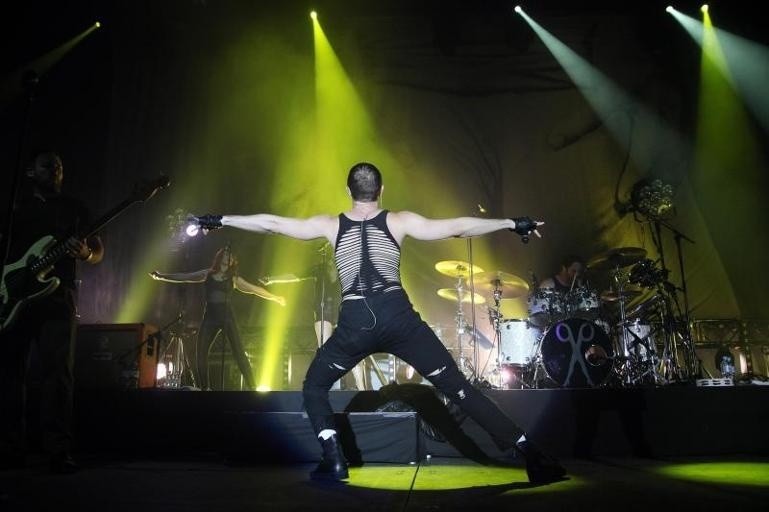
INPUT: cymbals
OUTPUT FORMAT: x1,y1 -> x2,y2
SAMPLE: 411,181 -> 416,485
466,271 -> 530,300
587,247 -> 647,271
435,260 -> 483,279
437,287 -> 488,304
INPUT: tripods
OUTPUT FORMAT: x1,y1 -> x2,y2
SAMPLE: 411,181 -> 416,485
604,217 -> 712,388
451,278 -> 533,387
154,245 -> 198,392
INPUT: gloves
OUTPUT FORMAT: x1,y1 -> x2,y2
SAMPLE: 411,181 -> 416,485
508,215 -> 538,238
192,212 -> 223,231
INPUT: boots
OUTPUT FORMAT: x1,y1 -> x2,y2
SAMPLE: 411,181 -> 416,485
309,432 -> 351,484
517,433 -> 570,489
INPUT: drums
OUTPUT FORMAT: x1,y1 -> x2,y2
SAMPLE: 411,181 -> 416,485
534,317 -> 614,389
614,323 -> 658,365
497,318 -> 540,369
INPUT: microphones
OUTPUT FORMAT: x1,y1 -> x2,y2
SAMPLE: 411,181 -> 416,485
551,114 -> 606,152
319,241 -> 331,252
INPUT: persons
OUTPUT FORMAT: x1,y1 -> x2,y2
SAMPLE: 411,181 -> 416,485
538,253 -> 584,290
149,247 -> 287,390
0,143 -> 104,477
258,242 -> 367,392
187,162 -> 568,486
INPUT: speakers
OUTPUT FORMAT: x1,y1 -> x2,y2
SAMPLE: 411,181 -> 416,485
75,321 -> 181,391
651,319 -> 769,380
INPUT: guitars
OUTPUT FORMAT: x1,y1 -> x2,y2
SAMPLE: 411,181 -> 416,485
0,174 -> 171,335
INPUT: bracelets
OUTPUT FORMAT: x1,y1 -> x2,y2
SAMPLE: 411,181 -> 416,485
83,249 -> 94,264
